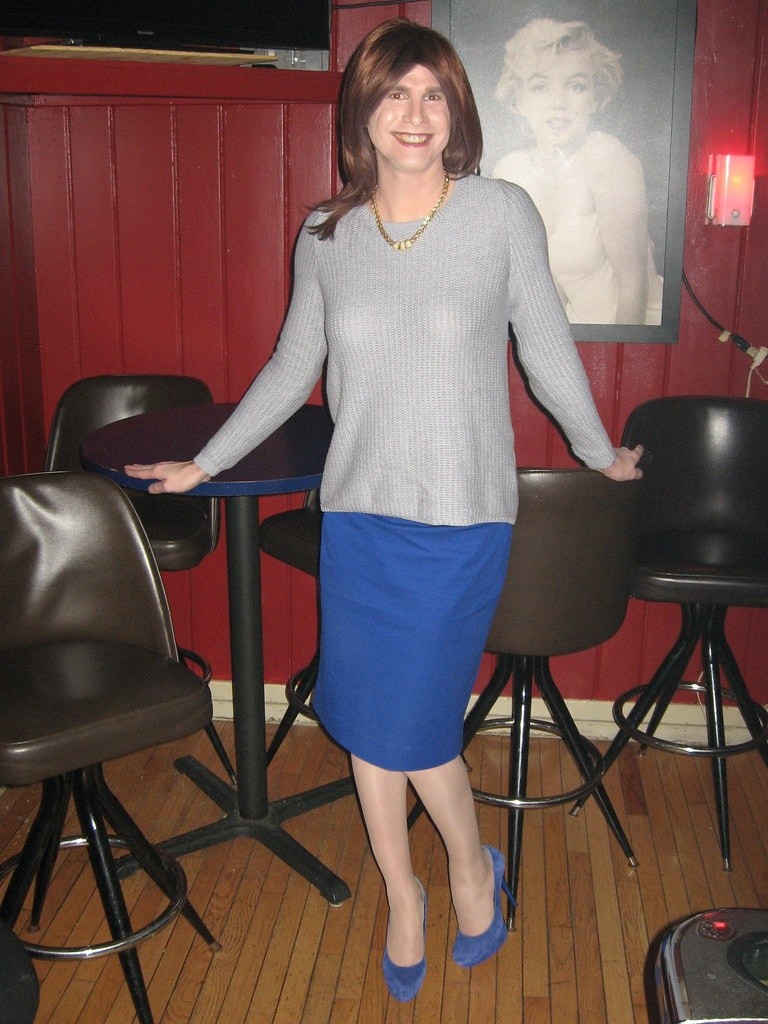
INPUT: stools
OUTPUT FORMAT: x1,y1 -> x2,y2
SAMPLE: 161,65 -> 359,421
261,488 -> 353,778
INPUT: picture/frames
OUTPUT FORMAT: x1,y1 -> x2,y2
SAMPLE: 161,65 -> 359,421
430,0 -> 698,345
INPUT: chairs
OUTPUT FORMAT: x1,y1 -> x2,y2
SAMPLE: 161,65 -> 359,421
404,467 -> 641,933
570,394 -> 768,872
44,373 -> 241,786
0,470 -> 225,1023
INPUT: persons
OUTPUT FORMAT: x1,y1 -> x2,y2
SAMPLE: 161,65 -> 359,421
123,19 -> 643,1001
490,20 -> 662,326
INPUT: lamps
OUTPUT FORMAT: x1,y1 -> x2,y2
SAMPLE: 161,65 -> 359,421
705,153 -> 756,226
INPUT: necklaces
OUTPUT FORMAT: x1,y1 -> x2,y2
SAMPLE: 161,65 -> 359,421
372,168 -> 448,252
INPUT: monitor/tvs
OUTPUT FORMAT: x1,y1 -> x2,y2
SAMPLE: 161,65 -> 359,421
0,0 -> 331,54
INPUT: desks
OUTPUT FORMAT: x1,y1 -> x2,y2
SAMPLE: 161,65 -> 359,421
82,404 -> 359,907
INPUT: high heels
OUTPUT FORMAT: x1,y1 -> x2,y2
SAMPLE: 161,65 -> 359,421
382,877 -> 427,1002
451,845 -> 519,967
